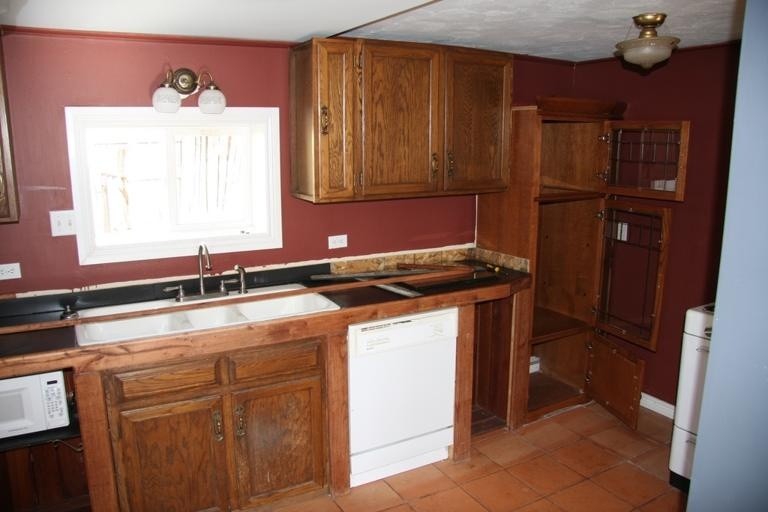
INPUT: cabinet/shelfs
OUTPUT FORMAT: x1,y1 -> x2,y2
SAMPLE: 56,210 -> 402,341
0,45 -> 22,224
359,38 -> 515,204
71,310 -> 353,511
475,105 -> 692,435
288,37 -> 357,205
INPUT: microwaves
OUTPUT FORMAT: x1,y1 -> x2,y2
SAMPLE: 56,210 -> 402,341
1,371 -> 72,441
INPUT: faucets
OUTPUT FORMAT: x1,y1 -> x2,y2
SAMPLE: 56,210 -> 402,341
196,242 -> 213,296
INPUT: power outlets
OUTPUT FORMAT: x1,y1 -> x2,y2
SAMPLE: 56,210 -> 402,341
0,262 -> 22,281
327,232 -> 348,250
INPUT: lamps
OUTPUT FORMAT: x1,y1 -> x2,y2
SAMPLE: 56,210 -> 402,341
151,65 -> 227,115
615,13 -> 679,71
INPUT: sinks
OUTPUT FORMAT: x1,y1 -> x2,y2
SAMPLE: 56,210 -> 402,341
74,298 -> 193,347
183,296 -> 250,329
231,283 -> 343,321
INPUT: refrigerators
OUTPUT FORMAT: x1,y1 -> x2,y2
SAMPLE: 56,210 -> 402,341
347,306 -> 458,488
